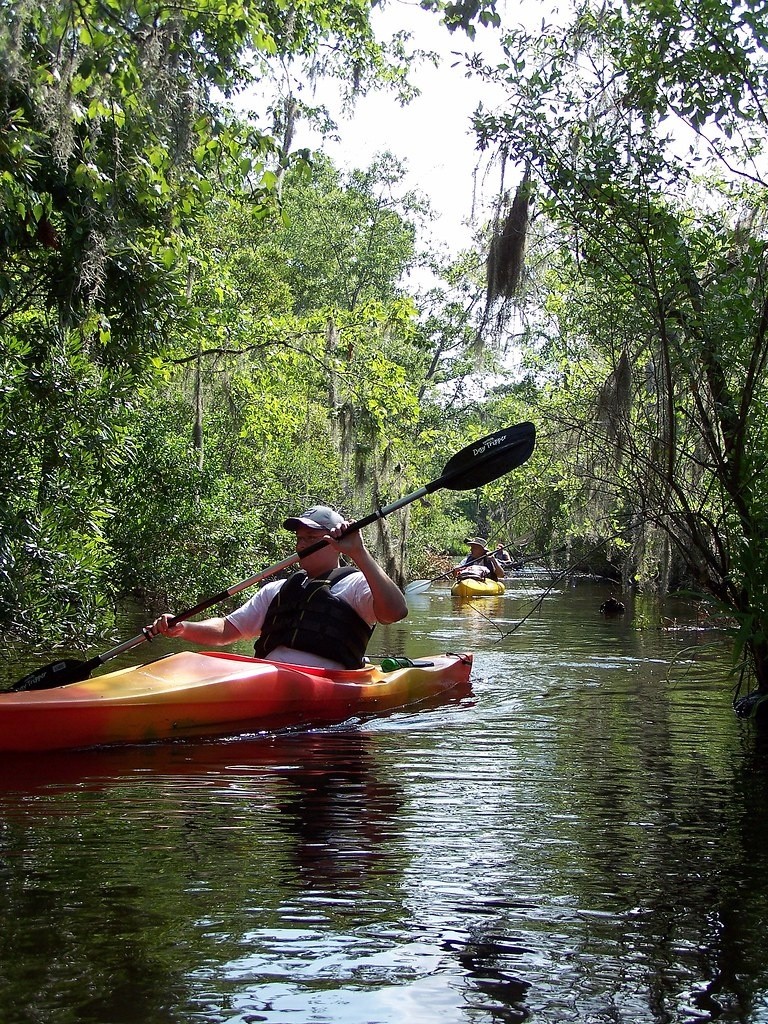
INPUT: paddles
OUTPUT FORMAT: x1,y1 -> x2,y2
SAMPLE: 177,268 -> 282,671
403,529 -> 537,595
2,420 -> 537,697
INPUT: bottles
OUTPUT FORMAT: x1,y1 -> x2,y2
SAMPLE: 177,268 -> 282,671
381,658 -> 415,673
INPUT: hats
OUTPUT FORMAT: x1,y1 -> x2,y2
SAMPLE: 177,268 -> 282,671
282,505 -> 345,531
467,537 -> 490,552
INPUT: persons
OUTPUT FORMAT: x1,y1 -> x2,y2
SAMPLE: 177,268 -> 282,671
145,504 -> 411,668
452,536 -> 505,582
493,544 -> 511,565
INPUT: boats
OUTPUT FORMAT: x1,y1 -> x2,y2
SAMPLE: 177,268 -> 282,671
450,577 -> 505,596
0,648 -> 476,753
